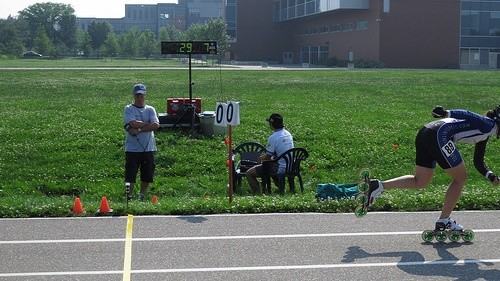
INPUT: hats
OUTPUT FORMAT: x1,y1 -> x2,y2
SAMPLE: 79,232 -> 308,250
132,84 -> 146,94
266,113 -> 283,124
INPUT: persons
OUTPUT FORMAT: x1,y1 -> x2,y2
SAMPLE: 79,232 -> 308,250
124,84 -> 160,200
245,113 -> 294,194
369,105 -> 500,227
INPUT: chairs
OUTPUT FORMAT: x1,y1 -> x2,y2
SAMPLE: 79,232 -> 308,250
232,142 -> 273,195
262,147 -> 309,194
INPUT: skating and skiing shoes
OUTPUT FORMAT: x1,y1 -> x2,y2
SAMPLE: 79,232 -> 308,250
421,217 -> 475,243
354,167 -> 384,218
